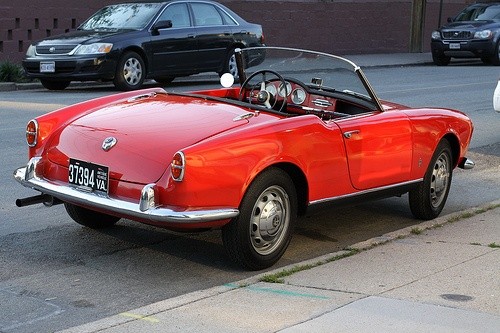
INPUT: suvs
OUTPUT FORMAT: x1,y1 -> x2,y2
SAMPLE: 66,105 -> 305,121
431,0 -> 500,68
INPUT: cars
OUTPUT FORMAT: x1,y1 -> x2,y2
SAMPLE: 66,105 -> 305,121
21,0 -> 267,91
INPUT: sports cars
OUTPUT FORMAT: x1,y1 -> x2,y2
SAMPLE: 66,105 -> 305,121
13,45 -> 476,271
